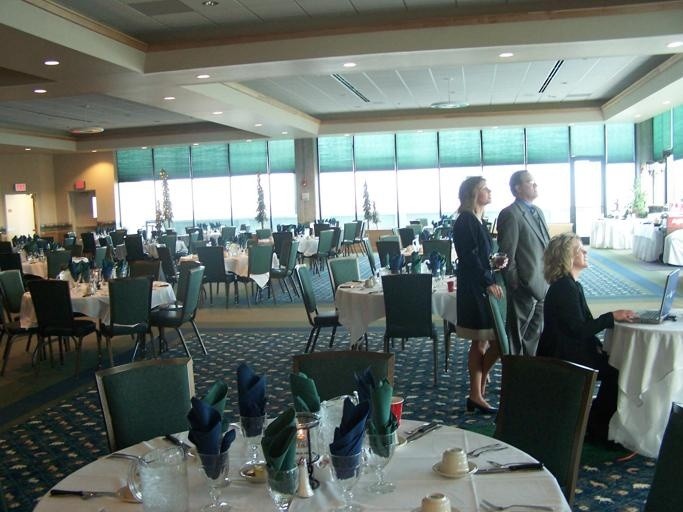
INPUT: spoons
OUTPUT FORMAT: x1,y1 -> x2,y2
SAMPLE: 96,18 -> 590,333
487,460 -> 541,468
405,424 -> 434,435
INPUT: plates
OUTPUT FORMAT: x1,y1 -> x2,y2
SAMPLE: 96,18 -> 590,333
411,506 -> 459,511
118,484 -> 143,504
431,462 -> 477,479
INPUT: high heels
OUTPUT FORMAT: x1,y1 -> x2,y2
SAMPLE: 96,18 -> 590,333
467,398 -> 499,413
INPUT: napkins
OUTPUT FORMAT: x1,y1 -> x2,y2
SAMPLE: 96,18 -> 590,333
363,375 -> 400,461
329,396 -> 373,480
184,377 -> 236,479
235,359 -> 268,440
288,371 -> 322,415
261,407 -> 299,494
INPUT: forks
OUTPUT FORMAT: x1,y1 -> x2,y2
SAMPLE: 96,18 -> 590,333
467,444 -> 508,459
479,500 -> 554,511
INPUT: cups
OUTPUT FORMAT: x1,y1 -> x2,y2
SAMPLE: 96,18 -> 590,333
443,448 -> 469,473
391,397 -> 405,423
423,494 -> 450,511
495,252 -> 508,268
448,282 -> 454,292
127,443 -> 188,511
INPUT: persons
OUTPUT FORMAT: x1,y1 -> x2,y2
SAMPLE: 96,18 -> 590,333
534,233 -> 640,449
496,171 -> 551,357
451,175 -> 510,414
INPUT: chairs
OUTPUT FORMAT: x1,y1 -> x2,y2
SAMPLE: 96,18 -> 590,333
94,357 -> 196,453
292,262 -> 342,353
2,219 -> 374,314
2,257 -> 210,378
493,354 -> 602,510
642,401 -> 682,512
489,286 -> 515,354
327,218 -> 458,387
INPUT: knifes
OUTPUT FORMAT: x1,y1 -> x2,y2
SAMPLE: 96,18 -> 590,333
478,464 -> 543,474
49,489 -> 118,499
406,422 -> 438,441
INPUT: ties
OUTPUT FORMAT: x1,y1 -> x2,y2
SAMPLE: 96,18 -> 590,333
530,207 -> 549,244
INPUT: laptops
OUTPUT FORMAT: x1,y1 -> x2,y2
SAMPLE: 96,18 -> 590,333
630,267 -> 680,325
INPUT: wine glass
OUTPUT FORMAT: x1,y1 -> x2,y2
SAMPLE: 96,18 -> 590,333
197,415 -> 395,511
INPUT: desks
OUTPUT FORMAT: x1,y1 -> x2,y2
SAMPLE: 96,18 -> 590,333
585,218 -> 631,251
30,411 -> 572,512
600,303 -> 682,461
628,217 -> 681,267
